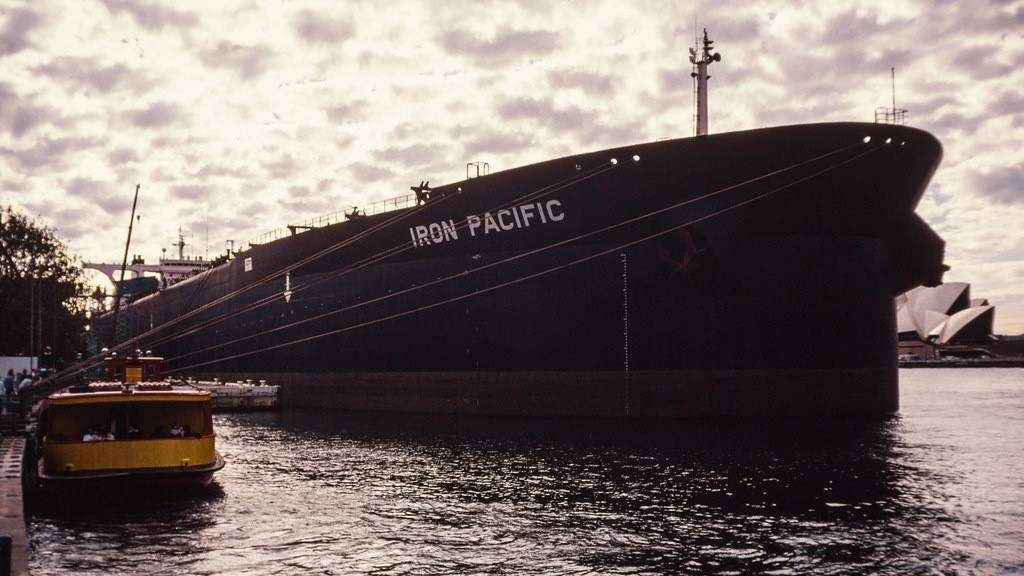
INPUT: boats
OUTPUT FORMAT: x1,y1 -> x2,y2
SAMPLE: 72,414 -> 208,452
28,350 -> 226,500
161,375 -> 282,409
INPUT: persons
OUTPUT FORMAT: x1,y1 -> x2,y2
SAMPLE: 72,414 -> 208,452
0,368 -> 40,415
170,422 -> 184,436
82,425 -> 115,441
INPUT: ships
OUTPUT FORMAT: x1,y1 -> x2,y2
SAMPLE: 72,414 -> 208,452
81,27 -> 952,421
